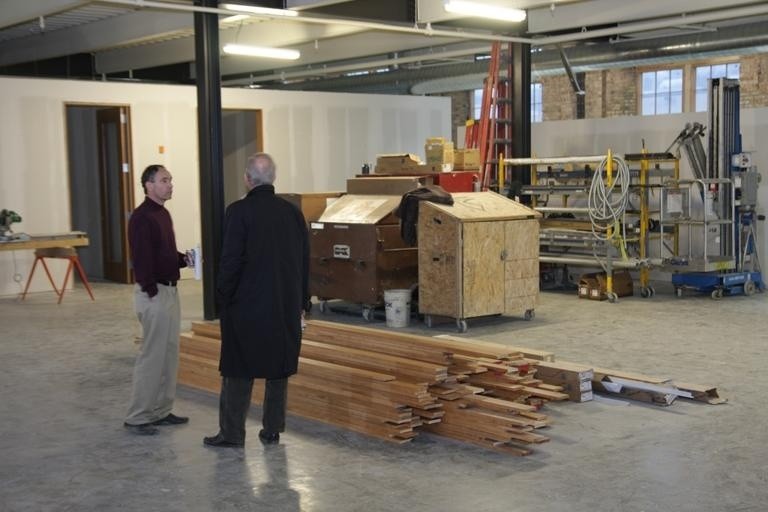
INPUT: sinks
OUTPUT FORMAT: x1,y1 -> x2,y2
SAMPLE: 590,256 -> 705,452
384,288 -> 411,328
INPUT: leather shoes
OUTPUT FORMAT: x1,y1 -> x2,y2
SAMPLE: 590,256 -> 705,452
204,434 -> 244,448
259,429 -> 279,442
124,421 -> 158,435
153,412 -> 188,425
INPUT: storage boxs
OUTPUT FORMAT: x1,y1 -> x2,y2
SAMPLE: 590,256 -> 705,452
455,149 -> 482,171
430,145 -> 456,172
588,273 -> 634,300
574,269 -> 610,299
376,154 -> 420,173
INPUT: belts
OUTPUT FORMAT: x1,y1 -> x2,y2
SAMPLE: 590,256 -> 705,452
159,279 -> 177,286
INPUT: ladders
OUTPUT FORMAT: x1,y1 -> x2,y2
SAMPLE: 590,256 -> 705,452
478,76 -> 508,184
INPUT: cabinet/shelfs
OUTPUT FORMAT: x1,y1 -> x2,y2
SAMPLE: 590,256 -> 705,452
418,189 -> 539,327
304,195 -> 417,318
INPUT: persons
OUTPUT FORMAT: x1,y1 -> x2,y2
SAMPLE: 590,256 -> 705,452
123,164 -> 195,436
203,152 -> 311,448
